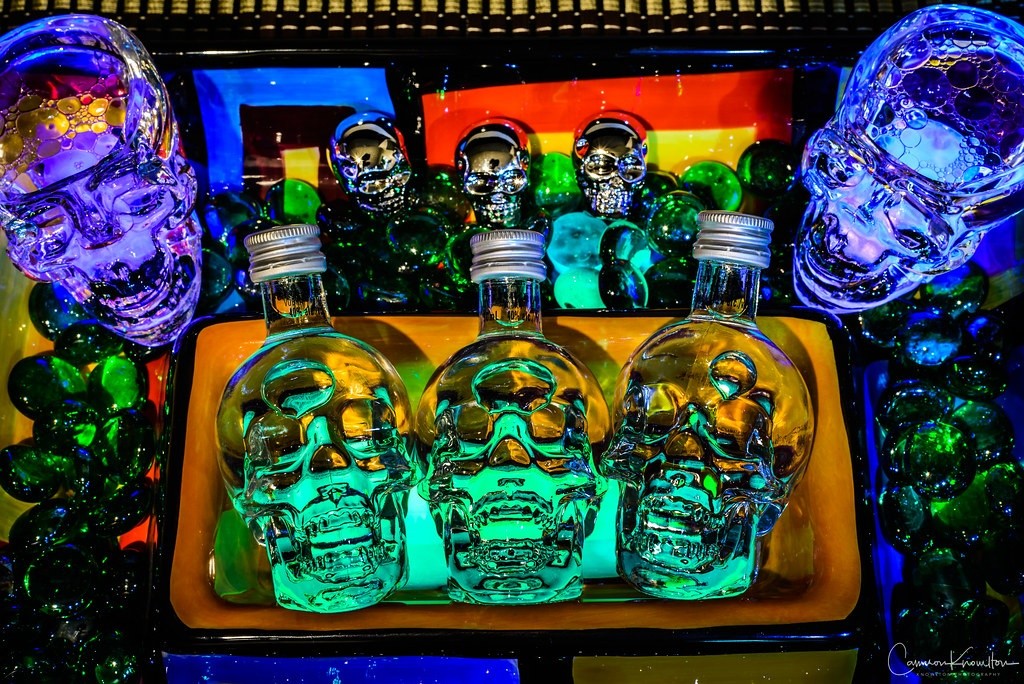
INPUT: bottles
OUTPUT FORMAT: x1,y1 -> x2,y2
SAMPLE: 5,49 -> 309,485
599,211 -> 810,598
414,232 -> 613,605
215,224 -> 423,613
